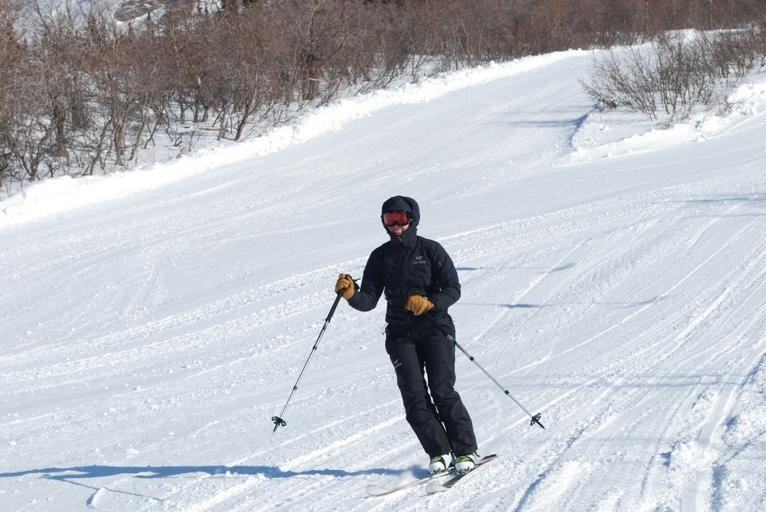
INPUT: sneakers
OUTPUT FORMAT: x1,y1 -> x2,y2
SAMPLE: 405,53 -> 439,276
429,452 -> 454,473
453,449 -> 481,472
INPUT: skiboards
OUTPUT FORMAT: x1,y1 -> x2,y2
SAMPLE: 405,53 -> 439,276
366,454 -> 498,496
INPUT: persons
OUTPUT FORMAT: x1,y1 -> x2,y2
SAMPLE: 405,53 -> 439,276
336,196 -> 477,472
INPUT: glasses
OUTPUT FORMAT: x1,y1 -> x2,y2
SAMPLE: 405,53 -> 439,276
382,210 -> 414,225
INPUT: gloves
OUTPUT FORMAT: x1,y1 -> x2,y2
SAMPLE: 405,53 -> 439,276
405,294 -> 434,317
334,272 -> 357,302
